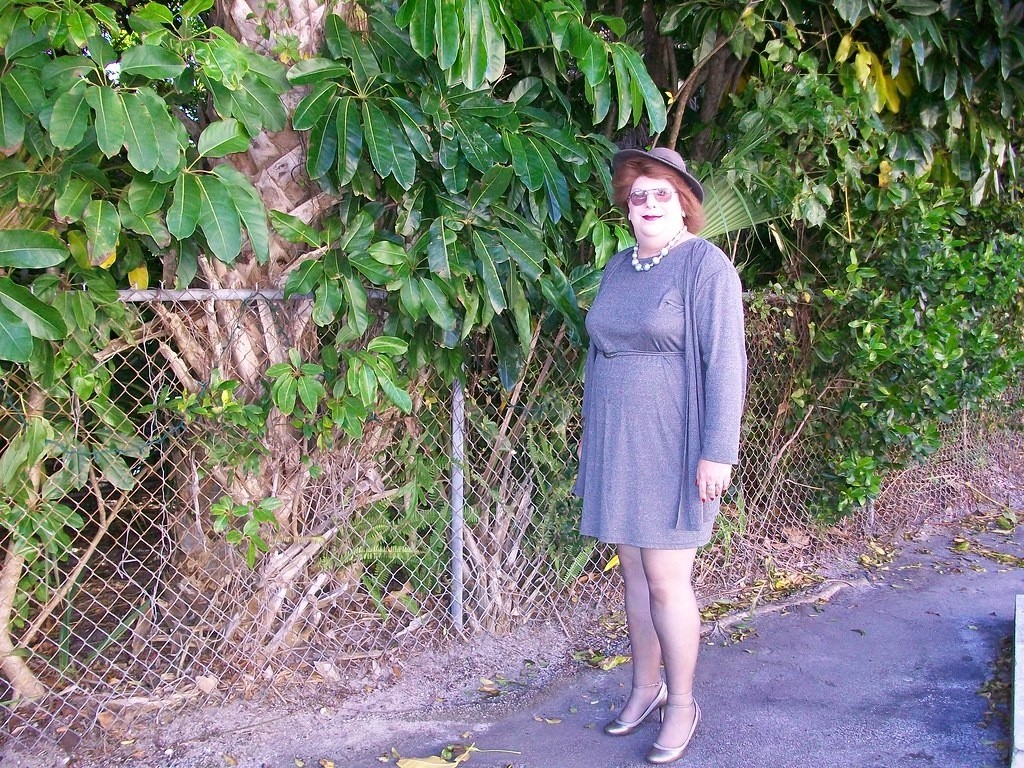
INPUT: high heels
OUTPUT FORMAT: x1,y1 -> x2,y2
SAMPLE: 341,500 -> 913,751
646,696 -> 701,764
603,678 -> 668,736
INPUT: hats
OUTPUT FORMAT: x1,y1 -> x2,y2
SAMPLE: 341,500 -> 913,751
612,147 -> 705,204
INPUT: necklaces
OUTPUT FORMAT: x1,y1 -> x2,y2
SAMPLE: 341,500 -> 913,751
632,225 -> 687,271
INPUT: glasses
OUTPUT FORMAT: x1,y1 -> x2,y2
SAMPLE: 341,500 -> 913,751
627,187 -> 676,206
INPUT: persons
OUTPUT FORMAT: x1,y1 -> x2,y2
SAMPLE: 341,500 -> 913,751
575,148 -> 747,764
